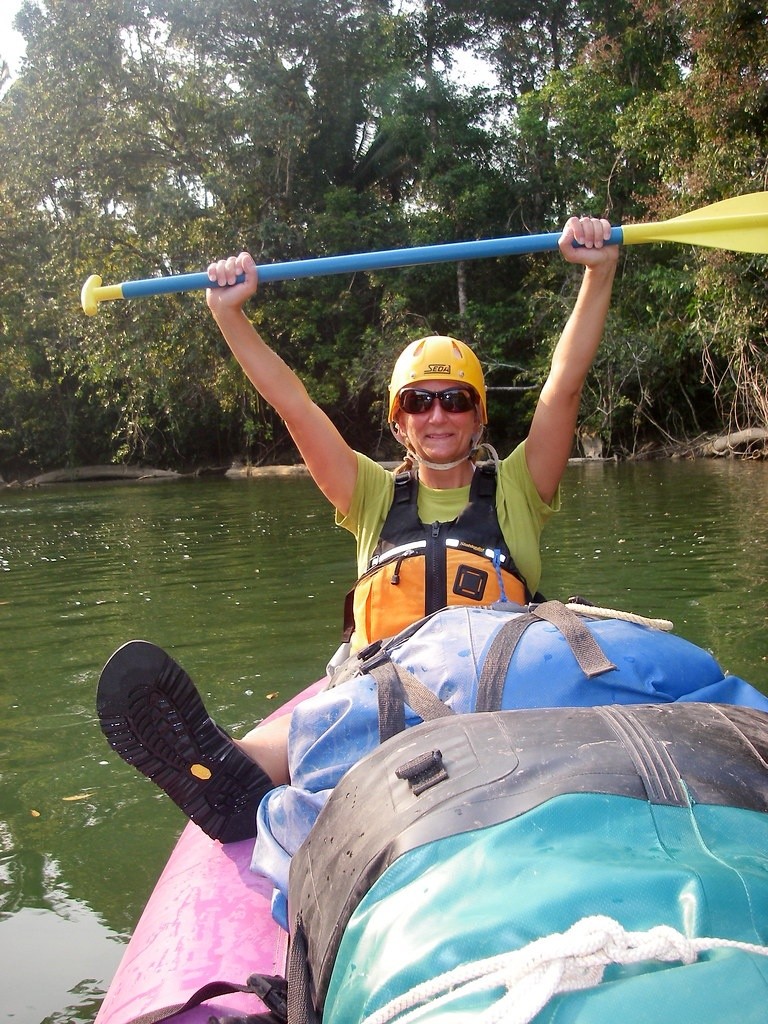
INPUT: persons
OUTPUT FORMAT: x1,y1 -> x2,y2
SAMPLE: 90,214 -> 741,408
95,216 -> 621,846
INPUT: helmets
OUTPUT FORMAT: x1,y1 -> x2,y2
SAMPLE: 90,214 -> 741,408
387,335 -> 488,425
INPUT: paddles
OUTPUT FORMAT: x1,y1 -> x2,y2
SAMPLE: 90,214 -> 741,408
76,178 -> 767,317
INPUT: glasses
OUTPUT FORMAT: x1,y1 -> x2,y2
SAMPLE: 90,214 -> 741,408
398,388 -> 478,413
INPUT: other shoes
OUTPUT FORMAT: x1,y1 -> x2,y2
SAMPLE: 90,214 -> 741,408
96,639 -> 276,844
